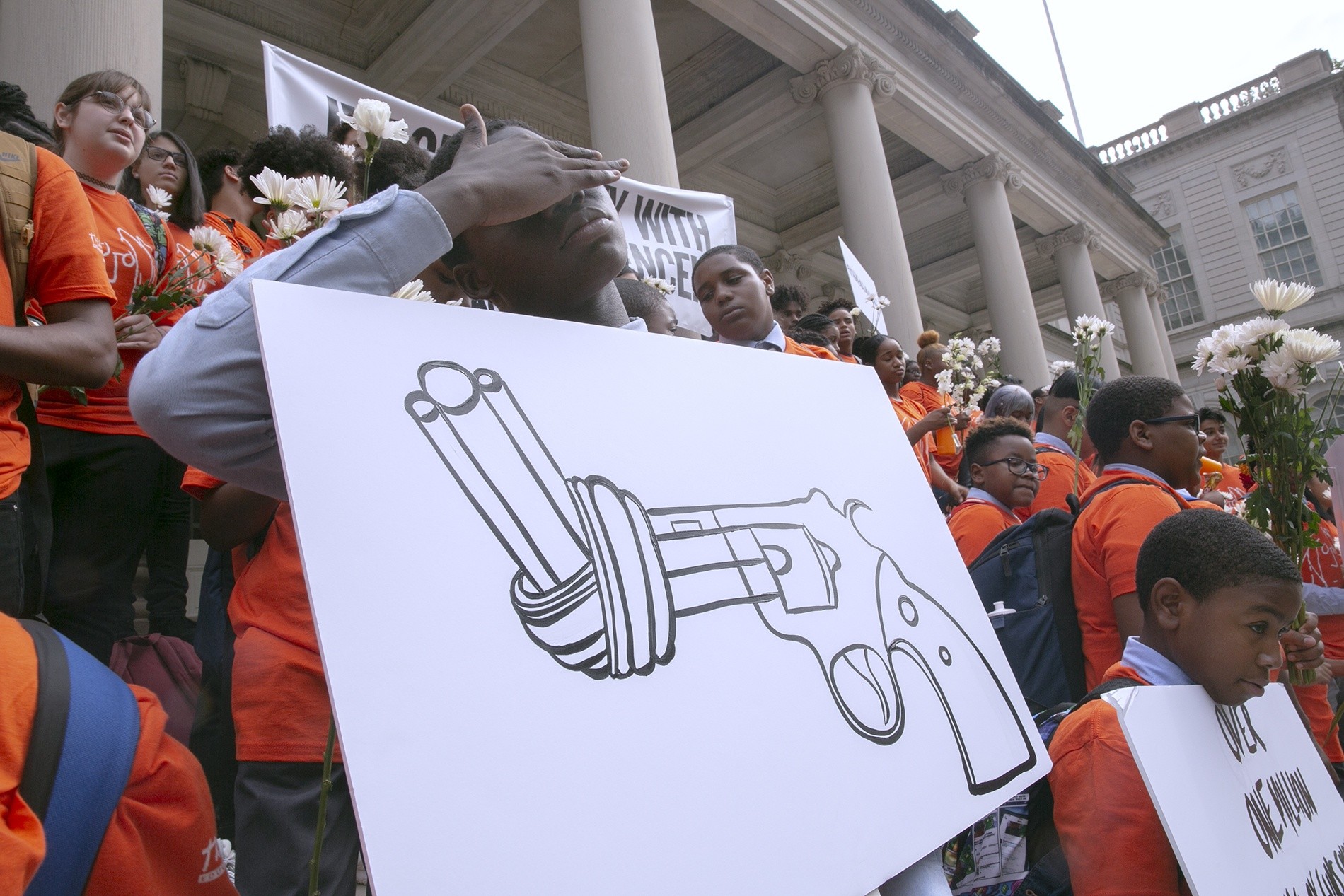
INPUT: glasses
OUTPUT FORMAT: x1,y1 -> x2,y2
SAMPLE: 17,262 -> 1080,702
145,146 -> 189,168
1143,414 -> 1201,435
980,457 -> 1050,481
70,91 -> 157,130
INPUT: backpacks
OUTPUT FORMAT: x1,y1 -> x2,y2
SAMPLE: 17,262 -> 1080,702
967,479 -> 1184,725
108,632 -> 202,750
941,677 -> 1183,896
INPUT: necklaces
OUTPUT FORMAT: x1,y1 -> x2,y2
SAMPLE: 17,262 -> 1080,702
74,170 -> 116,191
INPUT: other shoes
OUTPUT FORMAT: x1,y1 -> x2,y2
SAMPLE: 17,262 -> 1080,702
148,621 -> 196,646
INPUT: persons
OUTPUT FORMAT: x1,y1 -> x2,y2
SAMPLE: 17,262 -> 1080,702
0,69 -> 1344,896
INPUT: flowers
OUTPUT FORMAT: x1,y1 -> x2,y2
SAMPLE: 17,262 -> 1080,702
1069,314 -> 1115,496
1190,281 -> 1344,686
935,333 -> 1003,452
1040,359 -> 1074,395
33,191 -> 244,396
849,292 -> 890,334
249,96 -> 408,250
